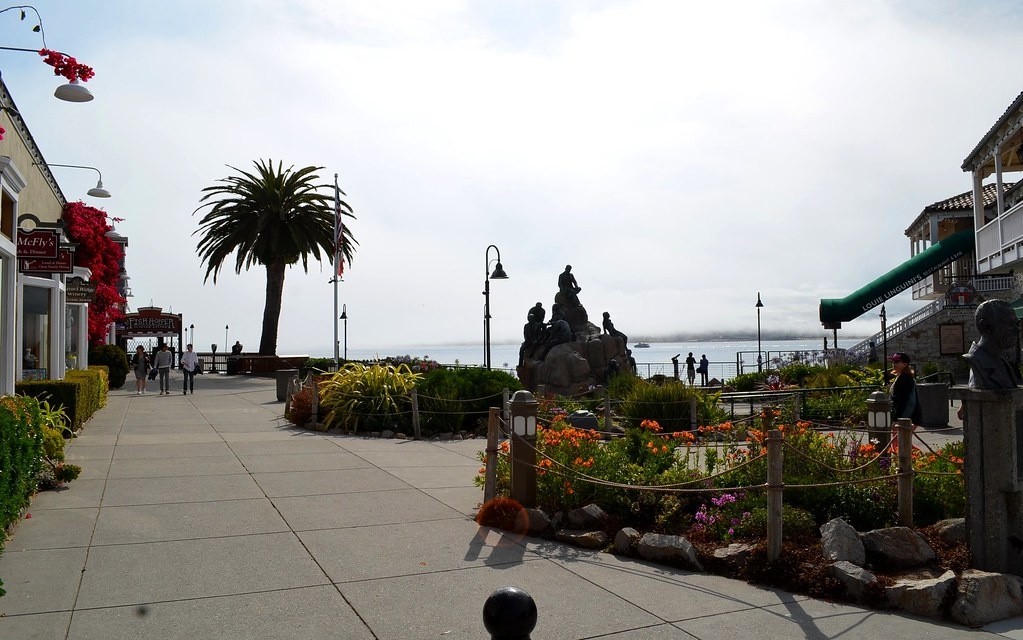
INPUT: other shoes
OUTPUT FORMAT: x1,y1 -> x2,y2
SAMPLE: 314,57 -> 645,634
160,391 -> 164,395
142,389 -> 145,394
166,391 -> 170,394
183,391 -> 186,395
138,391 -> 141,395
191,391 -> 193,394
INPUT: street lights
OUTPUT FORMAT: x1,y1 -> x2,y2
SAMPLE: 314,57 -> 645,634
755,292 -> 765,373
339,304 -> 348,363
190,324 -> 195,345
184,328 -> 188,352
485,245 -> 509,370
225,324 -> 229,352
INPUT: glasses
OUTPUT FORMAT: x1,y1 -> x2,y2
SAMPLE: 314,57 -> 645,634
891,360 -> 901,364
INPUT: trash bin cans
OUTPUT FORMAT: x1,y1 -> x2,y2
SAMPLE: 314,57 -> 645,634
227,358 -> 237,375
276,369 -> 300,402
911,383 -> 950,429
566,410 -> 598,431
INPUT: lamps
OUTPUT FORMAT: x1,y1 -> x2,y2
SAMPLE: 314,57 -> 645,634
327,358 -> 338,373
865,387 -> 893,446
506,388 -> 540,507
119,267 -> 130,279
169,305 -> 173,315
0,45 -> 94,102
149,298 -> 154,310
33,160 -> 112,199
106,216 -> 115,231
127,291 -> 134,297
123,280 -> 131,290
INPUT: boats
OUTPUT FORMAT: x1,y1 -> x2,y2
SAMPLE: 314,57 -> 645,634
634,343 -> 650,348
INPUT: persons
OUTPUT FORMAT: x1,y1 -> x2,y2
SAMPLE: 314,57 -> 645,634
181,344 -> 199,395
602,312 -> 636,380
961,299 -> 1021,389
558,265 -> 583,307
686,352 -> 696,387
231,341 -> 243,355
700,354 -> 708,387
517,303 -> 572,368
886,352 -> 922,452
131,345 -> 153,394
866,342 -> 877,364
154,343 -> 172,394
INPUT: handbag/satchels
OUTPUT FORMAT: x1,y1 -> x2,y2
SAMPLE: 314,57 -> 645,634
696,368 -> 701,373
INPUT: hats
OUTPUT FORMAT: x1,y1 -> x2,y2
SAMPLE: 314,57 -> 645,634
887,352 -> 911,365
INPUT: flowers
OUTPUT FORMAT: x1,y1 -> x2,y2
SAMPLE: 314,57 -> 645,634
62,201 -> 126,345
0,6 -> 95,81
